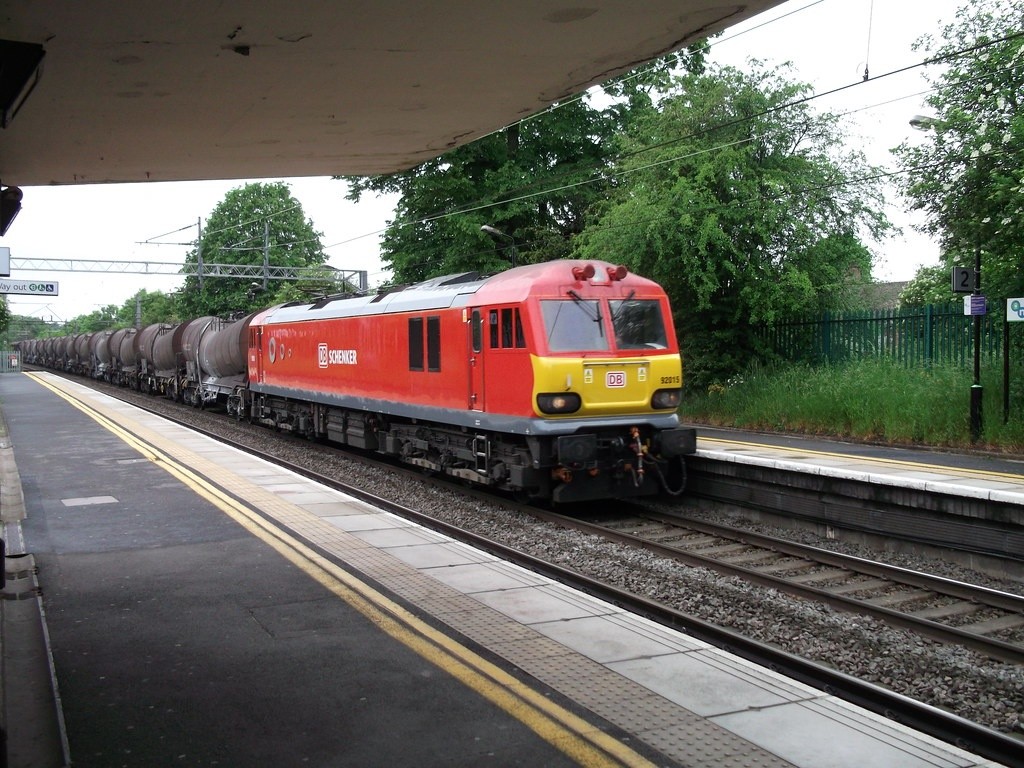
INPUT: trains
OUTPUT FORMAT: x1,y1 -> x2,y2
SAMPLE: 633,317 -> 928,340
11,259 -> 697,513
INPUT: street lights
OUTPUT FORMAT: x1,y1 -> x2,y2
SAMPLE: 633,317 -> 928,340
480,225 -> 514,268
909,115 -> 984,444
